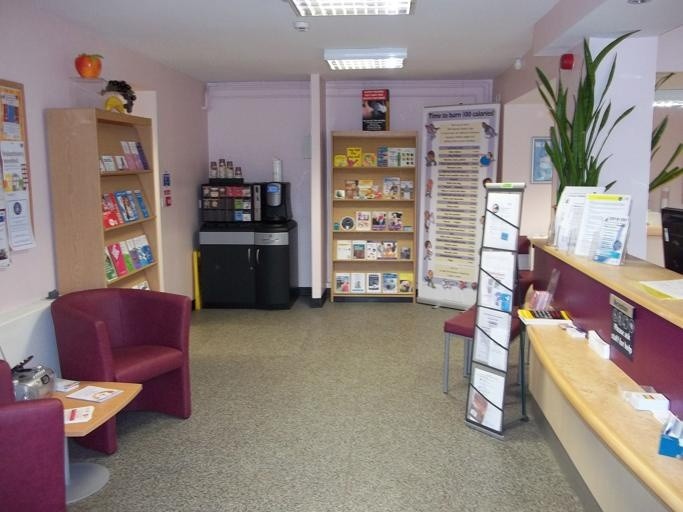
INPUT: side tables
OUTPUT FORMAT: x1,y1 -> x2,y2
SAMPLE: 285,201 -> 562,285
43,378 -> 143,505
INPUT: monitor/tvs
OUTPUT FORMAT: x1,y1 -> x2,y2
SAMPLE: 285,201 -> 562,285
660,207 -> 683,275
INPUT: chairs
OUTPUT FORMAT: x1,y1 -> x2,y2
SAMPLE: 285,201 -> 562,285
441,236 -> 532,423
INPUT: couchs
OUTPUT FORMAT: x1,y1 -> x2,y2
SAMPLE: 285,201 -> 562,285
51,288 -> 193,456
0,358 -> 69,512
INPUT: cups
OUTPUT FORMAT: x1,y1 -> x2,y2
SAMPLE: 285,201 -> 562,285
270,157 -> 281,182
207,158 -> 242,179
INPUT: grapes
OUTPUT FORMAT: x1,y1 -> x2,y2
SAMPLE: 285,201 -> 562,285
104,80 -> 136,114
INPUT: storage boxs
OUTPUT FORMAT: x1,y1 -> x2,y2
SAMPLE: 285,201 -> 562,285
69,77 -> 108,107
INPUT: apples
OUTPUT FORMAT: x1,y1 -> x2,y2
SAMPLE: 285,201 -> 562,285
76,52 -> 104,77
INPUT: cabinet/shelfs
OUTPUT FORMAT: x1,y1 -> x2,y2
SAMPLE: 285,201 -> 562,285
43,106 -> 161,300
197,219 -> 299,310
329,129 -> 418,305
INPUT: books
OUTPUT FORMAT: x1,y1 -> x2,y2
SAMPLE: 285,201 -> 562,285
333,146 -> 416,293
100,141 -> 156,291
517,309 -> 573,325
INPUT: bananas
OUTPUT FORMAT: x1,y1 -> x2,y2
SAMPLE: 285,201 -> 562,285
105,94 -> 127,114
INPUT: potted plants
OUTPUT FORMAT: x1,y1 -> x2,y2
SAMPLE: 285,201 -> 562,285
533,28 -> 642,212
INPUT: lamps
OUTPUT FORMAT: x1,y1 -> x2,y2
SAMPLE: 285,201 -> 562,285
321,47 -> 407,73
286,0 -> 411,18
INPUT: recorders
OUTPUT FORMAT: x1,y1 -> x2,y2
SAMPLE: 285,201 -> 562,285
10,355 -> 54,400
